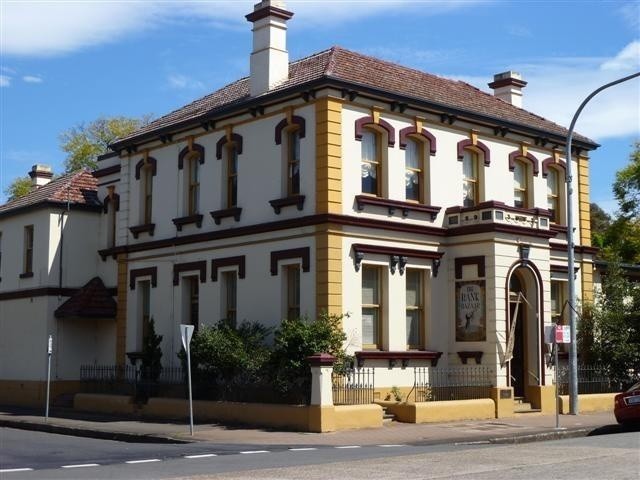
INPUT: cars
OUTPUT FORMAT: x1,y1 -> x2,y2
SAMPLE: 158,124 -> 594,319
612,382 -> 639,434
